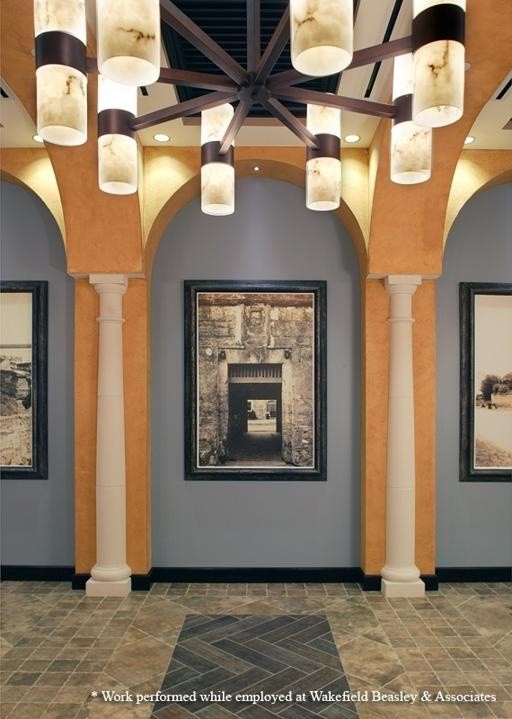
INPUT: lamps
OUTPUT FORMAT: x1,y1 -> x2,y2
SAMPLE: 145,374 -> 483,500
33,0 -> 466,217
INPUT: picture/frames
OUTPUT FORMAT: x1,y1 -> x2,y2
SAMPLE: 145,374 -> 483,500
182,279 -> 328,482
457,281 -> 512,482
0,279 -> 48,479
